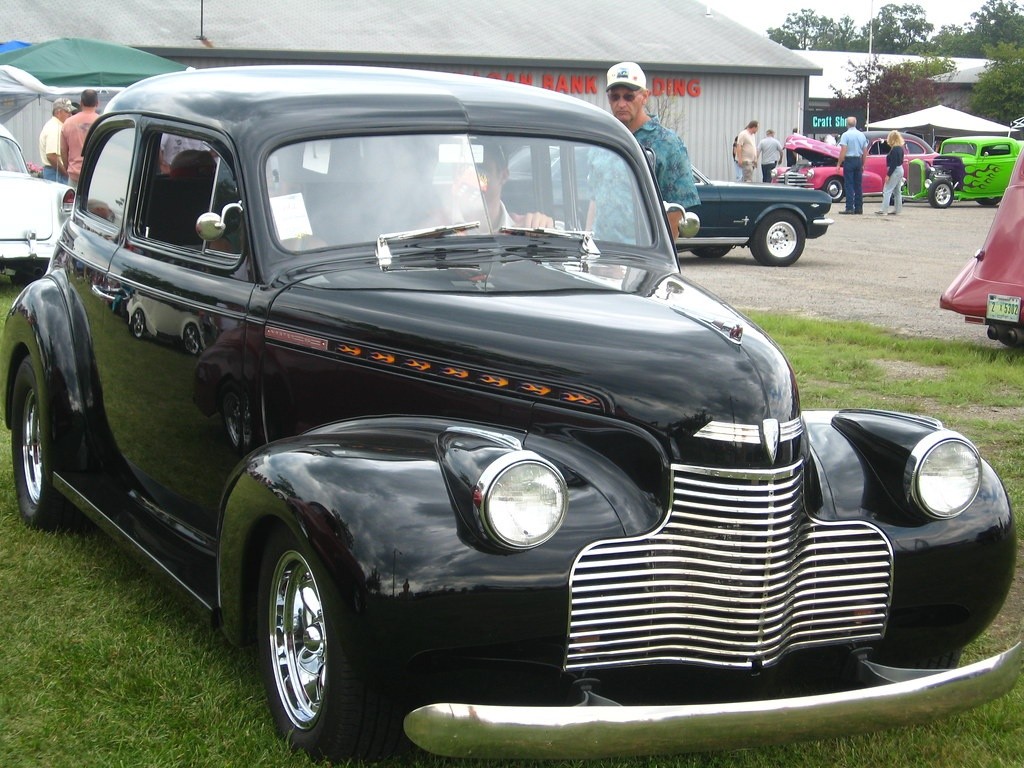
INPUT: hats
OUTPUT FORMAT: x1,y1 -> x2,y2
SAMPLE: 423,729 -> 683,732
606,62 -> 647,92
766,129 -> 775,134
53,98 -> 78,113
793,128 -> 798,132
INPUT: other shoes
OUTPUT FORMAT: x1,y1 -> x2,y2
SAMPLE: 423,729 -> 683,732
874,210 -> 887,216
888,212 -> 902,216
839,209 -> 855,214
854,206 -> 863,214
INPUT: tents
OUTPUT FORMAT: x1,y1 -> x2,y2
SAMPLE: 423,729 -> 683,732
0,36 -> 186,86
865,105 -> 1020,151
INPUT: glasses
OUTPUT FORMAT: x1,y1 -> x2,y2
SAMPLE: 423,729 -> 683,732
607,91 -> 642,101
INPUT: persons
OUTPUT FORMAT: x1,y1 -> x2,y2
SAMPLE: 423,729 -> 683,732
38,89 -> 219,188
732,117 -> 905,216
585,62 -> 702,244
210,139 -> 566,255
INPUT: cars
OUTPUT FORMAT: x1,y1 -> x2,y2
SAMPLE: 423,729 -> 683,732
1,126 -> 77,281
771,131 -> 938,206
1,63 -> 1022,767
481,141 -> 835,267
901,135 -> 1022,210
